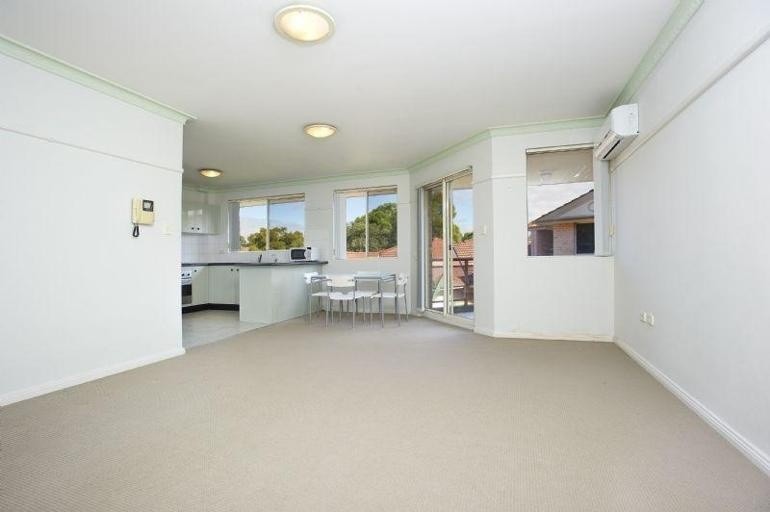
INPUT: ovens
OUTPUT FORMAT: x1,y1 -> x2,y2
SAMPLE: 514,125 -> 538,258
181,271 -> 193,304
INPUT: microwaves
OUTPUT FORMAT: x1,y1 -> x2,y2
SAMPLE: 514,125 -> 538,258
289,246 -> 317,262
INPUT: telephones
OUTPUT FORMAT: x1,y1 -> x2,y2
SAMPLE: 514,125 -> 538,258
132,197 -> 154,224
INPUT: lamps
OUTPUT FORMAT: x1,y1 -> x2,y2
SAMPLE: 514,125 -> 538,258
199,169 -> 224,178
304,124 -> 337,138
274,4 -> 336,47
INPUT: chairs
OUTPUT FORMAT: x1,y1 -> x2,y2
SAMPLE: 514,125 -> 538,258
304,272 -> 408,328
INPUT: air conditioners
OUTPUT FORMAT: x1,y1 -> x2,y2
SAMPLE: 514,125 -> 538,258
594,103 -> 639,161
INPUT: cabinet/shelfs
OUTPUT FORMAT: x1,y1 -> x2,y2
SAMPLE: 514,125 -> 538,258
182,203 -> 221,235
208,266 -> 239,311
192,266 -> 209,313
